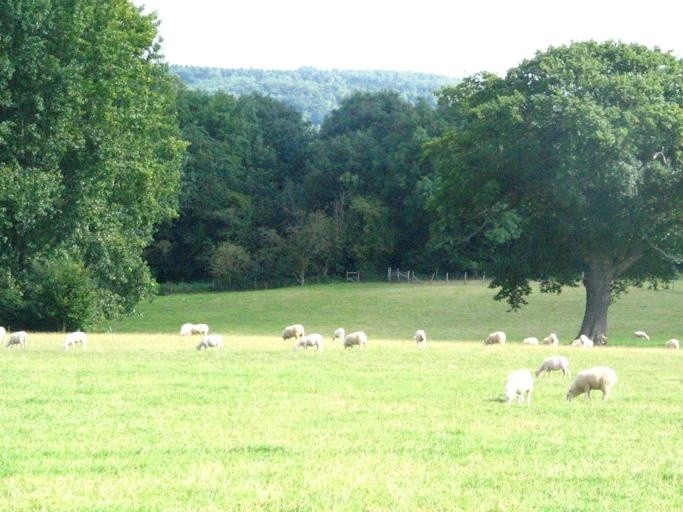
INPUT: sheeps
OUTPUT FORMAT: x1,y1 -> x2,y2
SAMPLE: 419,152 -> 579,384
543,334 -> 558,345
281,324 -> 325,352
331,327 -> 369,349
413,330 -> 426,346
179,323 -> 209,336
634,331 -> 649,340
523,337 -> 539,345
196,334 -> 225,351
503,368 -> 534,404
483,331 -> 507,345
565,366 -> 618,402
534,356 -> 569,377
572,335 -> 594,346
664,338 -> 680,349
63,331 -> 88,351
